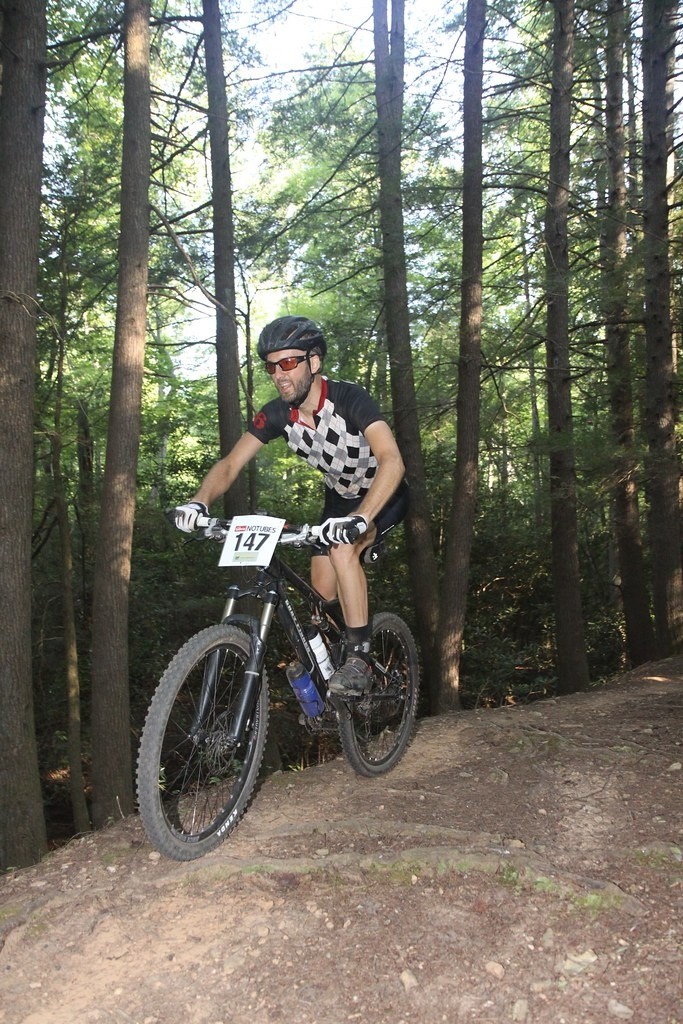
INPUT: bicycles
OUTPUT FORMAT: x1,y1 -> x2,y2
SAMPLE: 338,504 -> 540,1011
137,507 -> 424,859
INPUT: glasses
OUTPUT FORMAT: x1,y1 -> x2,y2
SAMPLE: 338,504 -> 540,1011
265,354 -> 319,375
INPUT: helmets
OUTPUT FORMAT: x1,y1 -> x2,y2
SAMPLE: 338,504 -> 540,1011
257,316 -> 327,361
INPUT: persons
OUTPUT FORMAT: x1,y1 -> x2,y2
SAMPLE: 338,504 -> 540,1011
173,316 -> 411,697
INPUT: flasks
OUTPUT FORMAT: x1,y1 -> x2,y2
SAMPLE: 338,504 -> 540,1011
302,620 -> 335,682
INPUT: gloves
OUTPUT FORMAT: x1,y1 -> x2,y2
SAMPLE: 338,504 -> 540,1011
174,501 -> 210,533
318,514 -> 367,546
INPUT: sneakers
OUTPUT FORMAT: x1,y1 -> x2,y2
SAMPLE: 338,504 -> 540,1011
328,656 -> 373,696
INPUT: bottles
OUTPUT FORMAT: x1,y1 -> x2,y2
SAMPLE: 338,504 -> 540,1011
287,659 -> 325,718
